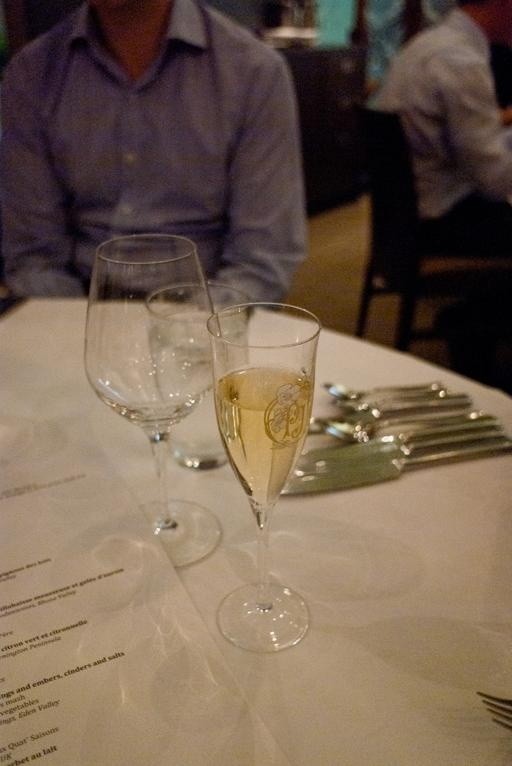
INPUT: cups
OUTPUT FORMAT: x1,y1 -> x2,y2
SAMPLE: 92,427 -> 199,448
142,280 -> 251,473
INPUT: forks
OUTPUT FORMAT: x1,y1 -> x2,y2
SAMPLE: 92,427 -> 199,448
475,690 -> 511,731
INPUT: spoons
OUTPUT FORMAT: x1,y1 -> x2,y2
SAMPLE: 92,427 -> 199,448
313,378 -> 489,443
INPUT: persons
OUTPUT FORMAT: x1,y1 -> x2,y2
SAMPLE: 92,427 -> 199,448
369,2 -> 511,367
3,0 -> 307,312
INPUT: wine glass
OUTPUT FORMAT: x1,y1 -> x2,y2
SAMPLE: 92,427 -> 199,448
204,300 -> 324,655
82,234 -> 221,569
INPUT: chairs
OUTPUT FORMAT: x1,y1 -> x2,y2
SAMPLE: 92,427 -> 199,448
354,108 -> 511,355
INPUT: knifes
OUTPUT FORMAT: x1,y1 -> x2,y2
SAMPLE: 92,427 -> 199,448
279,423 -> 510,501
